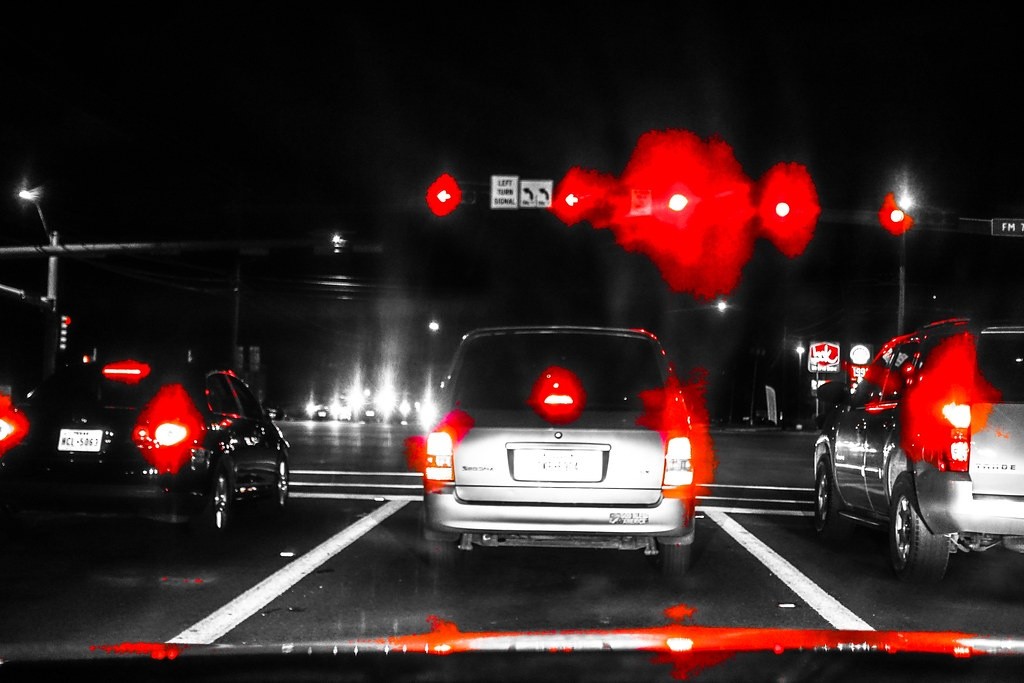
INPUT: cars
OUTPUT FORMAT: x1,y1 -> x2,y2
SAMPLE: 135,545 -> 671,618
814,318 -> 1024,584
422,325 -> 695,583
0,346 -> 290,532
